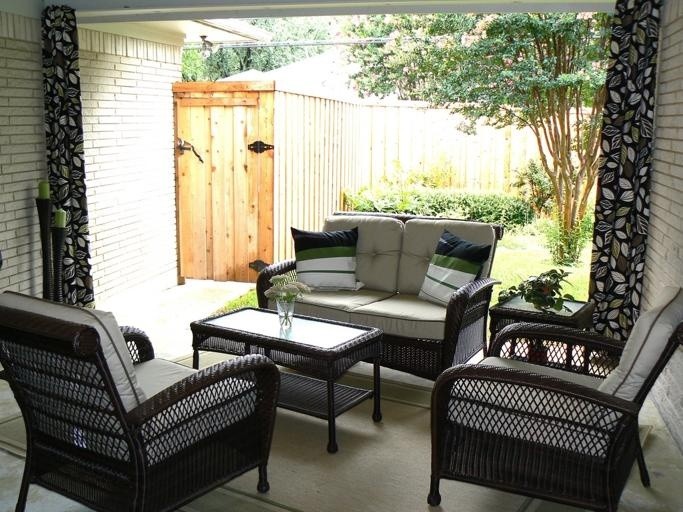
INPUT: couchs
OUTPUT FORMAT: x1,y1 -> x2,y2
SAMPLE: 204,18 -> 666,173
257,213 -> 503,381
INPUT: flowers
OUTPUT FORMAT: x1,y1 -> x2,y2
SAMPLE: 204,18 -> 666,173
263,274 -> 311,327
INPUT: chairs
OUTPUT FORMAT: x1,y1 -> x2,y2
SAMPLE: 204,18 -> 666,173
427,286 -> 683,512
0,290 -> 281,512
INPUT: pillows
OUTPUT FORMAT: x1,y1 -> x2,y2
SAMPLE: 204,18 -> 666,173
417,229 -> 492,307
291,226 -> 366,292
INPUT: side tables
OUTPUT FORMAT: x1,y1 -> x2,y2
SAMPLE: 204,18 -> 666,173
489,293 -> 594,373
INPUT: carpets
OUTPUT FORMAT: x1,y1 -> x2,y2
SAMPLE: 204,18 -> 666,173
0,351 -> 653,512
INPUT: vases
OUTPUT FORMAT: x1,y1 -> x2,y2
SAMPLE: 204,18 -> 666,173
528,343 -> 548,365
276,299 -> 295,329
